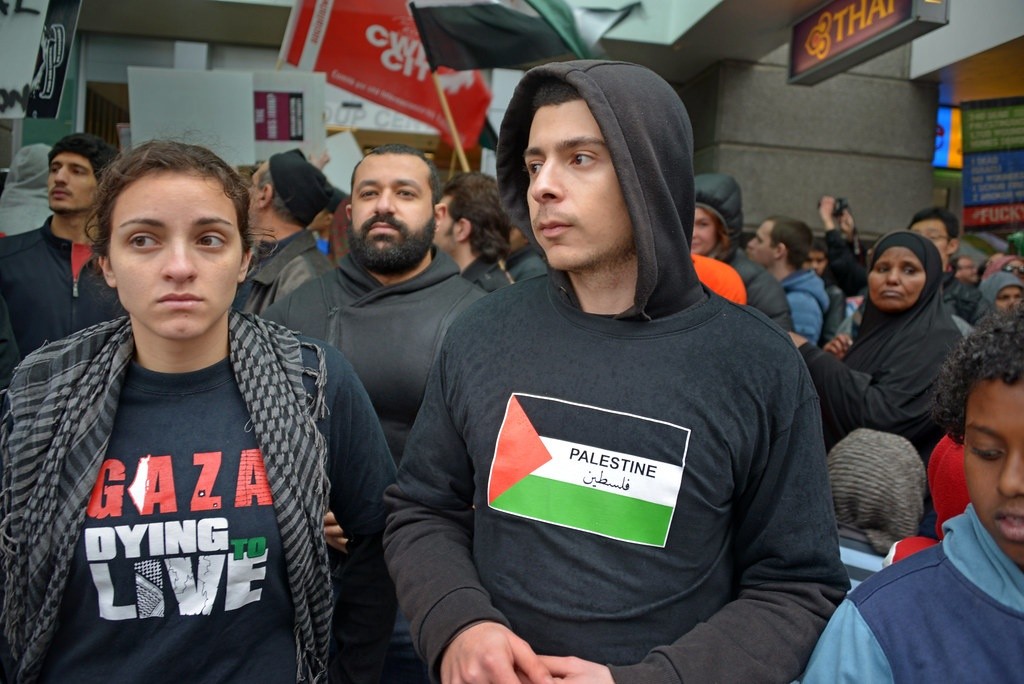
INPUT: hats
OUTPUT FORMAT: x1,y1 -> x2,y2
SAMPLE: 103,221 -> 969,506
269,147 -> 328,228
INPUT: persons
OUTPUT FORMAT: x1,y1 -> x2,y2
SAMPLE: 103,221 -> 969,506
381,59 -> 852,683
801,303 -> 1024,683
431,170 -> 512,292
231,147 -> 336,317
691,173 -> 1024,538
259,141 -> 493,684
307,188 -> 348,256
882,432 -> 973,568
503,225 -> 549,282
792,425 -> 926,684
0,128 -> 400,684
0,131 -> 129,362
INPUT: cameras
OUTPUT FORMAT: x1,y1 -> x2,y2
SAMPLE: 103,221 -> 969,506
817,197 -> 848,216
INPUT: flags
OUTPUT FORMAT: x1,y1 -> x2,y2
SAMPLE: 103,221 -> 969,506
276,1 -> 493,152
524,0 -> 644,59
408,1 -> 568,75
477,115 -> 500,157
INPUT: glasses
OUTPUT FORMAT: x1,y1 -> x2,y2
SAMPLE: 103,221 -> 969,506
926,233 -> 951,242
1002,265 -> 1024,274
